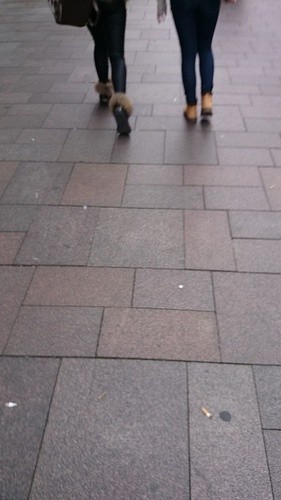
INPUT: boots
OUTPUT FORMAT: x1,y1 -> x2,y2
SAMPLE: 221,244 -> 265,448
95,80 -> 112,105
201,92 -> 213,115
108,93 -> 131,136
183,103 -> 197,123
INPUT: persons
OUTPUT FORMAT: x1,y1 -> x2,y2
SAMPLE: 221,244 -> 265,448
156,0 -> 236,120
47,0 -> 132,134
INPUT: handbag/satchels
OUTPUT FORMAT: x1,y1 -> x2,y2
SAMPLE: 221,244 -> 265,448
48,0 -> 94,28
